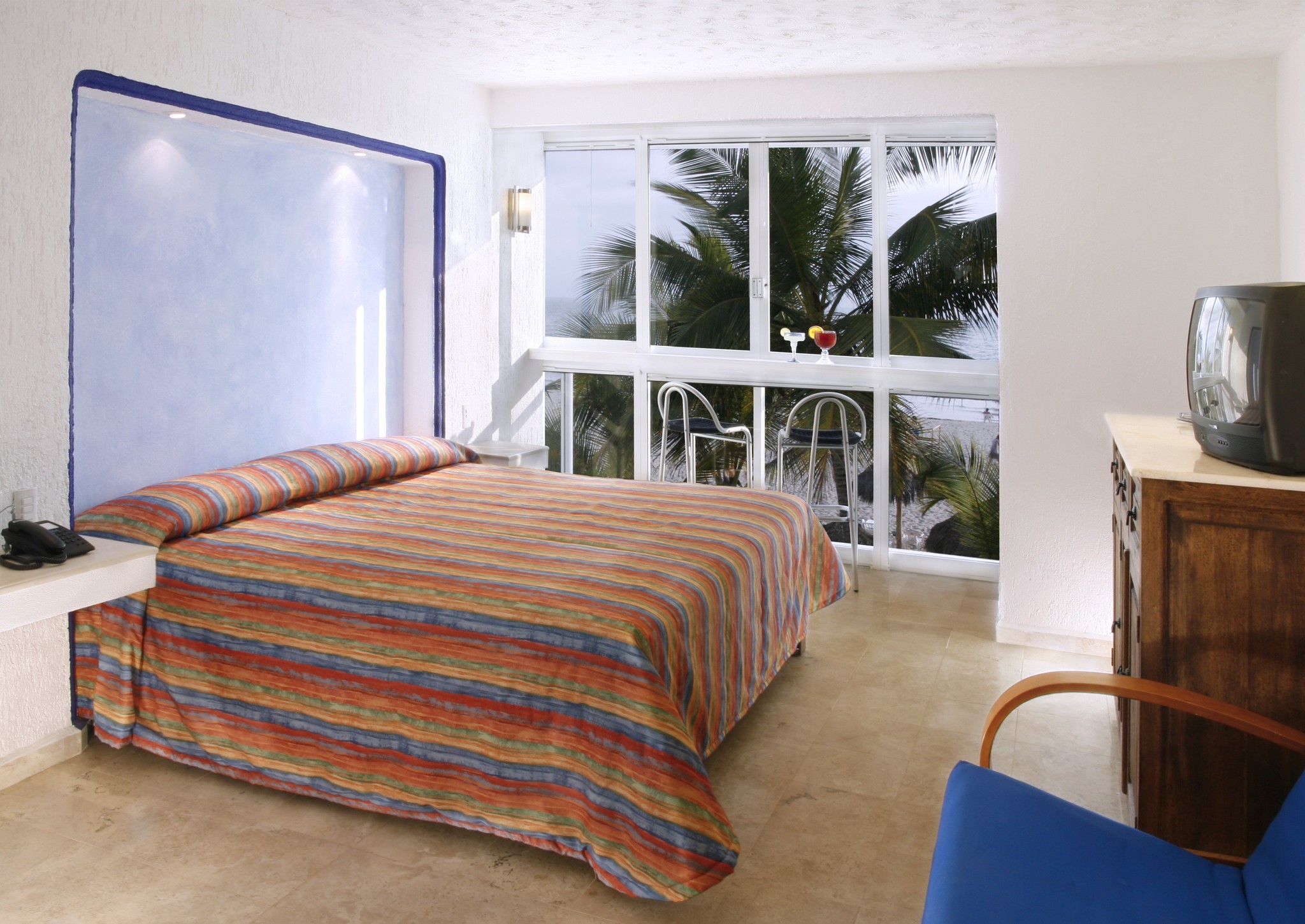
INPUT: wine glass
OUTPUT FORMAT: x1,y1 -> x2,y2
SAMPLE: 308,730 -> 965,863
814,330 -> 837,364
784,332 -> 805,363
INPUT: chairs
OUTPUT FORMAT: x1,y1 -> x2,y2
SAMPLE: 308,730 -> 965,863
920,671 -> 1304,923
653,382 -> 751,486
775,391 -> 869,593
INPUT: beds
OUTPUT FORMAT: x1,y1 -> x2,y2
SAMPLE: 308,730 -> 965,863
66,464 -> 851,907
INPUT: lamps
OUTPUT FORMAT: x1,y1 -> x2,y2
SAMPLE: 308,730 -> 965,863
508,184 -> 532,233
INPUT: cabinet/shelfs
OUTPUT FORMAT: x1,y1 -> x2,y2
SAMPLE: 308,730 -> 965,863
1102,415 -> 1304,860
0,525 -> 163,635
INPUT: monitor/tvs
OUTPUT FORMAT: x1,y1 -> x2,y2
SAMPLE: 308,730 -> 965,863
1187,281 -> 1305,475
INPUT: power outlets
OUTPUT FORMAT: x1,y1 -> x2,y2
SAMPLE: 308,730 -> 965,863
12,487 -> 38,526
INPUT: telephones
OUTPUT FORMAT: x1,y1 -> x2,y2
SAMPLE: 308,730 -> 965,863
1,518 -> 96,561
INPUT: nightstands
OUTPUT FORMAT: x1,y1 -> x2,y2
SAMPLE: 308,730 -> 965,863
463,440 -> 550,472
